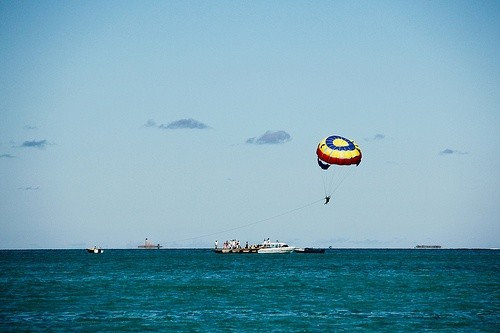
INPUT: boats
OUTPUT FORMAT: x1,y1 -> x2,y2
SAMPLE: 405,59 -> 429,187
87,248 -> 104,254
257,242 -> 297,254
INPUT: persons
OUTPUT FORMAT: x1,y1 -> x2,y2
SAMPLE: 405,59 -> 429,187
214,238 -> 279,249
